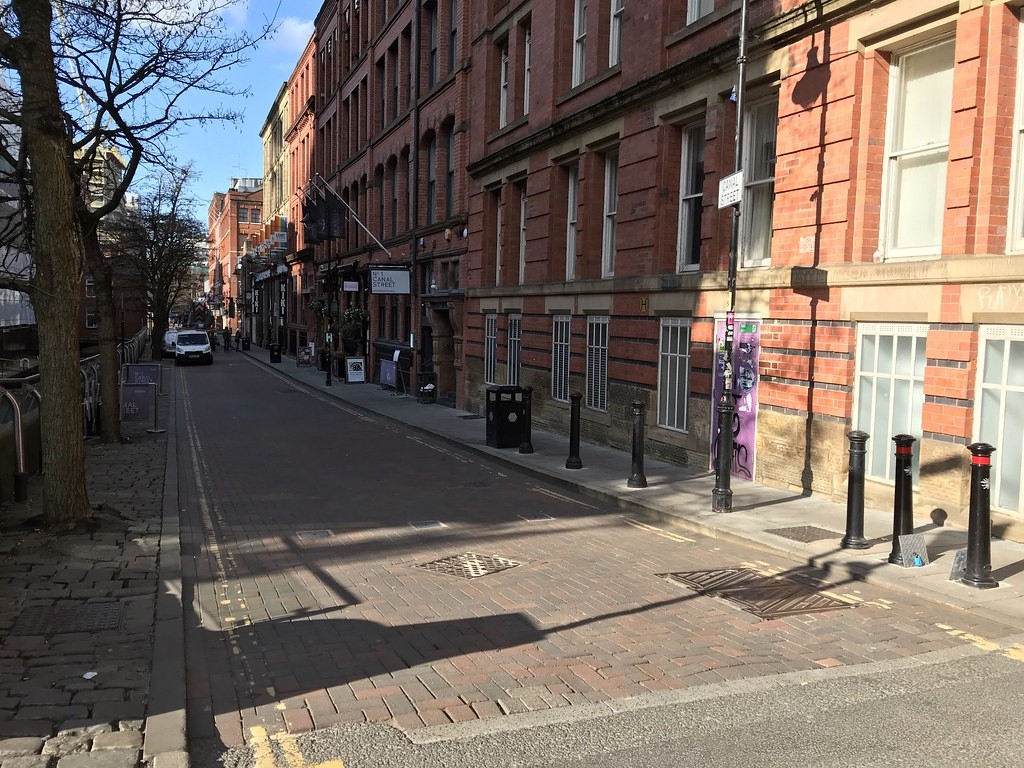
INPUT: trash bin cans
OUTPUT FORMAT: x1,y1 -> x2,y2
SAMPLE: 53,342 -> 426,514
270,343 -> 281,363
486,385 -> 524,448
241,336 -> 250,351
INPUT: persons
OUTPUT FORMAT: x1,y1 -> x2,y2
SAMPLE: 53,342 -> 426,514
223,326 -> 229,352
235,327 -> 242,352
209,329 -> 217,351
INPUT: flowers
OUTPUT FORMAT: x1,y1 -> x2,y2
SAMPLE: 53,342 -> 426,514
309,298 -> 323,312
344,306 -> 370,325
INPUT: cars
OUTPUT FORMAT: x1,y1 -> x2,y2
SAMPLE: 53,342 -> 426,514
171,328 -> 213,366
162,330 -> 178,354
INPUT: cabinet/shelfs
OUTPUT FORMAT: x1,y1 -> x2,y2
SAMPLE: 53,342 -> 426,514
416,371 -> 438,403
